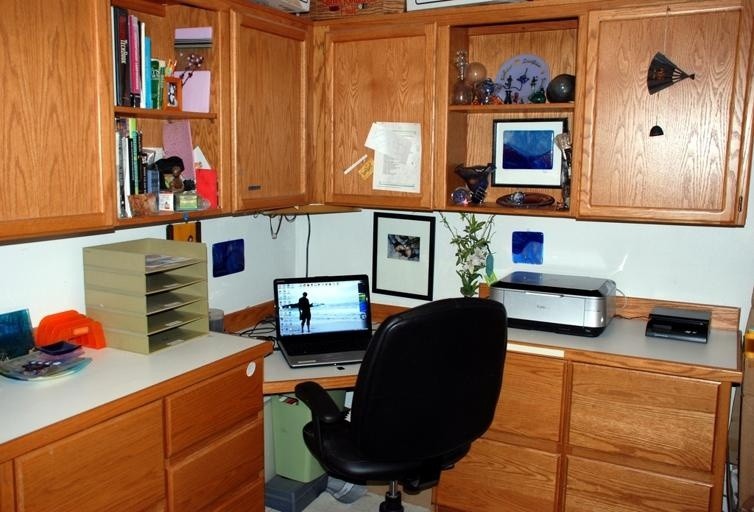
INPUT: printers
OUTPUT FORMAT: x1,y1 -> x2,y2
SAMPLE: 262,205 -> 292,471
489,271 -> 616,337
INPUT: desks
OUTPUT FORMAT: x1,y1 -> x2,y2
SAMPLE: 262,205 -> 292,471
229,315 -> 434,511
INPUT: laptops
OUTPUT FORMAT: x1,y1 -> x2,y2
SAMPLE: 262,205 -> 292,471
274,274 -> 372,368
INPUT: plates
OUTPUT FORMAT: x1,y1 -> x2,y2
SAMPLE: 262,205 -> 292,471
495,54 -> 550,105
496,191 -> 555,208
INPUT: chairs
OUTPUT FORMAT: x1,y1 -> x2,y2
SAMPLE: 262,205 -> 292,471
295,297 -> 507,511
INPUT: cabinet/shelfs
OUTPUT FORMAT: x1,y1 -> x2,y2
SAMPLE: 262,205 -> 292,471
445,17 -> 579,213
0,0 -> 115,242
2,397 -> 164,512
231,10 -> 313,211
432,338 -> 570,512
325,24 -> 437,209
562,349 -> 743,512
166,342 -> 273,511
579,2 -> 753,227
115,1 -> 232,227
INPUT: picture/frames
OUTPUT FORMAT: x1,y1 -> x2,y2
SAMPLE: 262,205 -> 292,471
372,213 -> 433,299
493,119 -> 567,187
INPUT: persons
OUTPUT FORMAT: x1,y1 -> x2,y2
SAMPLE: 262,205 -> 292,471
298,292 -> 314,333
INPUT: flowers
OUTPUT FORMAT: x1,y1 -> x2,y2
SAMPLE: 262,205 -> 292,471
440,210 -> 498,297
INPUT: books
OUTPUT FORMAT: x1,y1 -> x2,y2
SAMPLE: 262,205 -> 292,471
113,116 -> 160,219
111,6 -> 166,109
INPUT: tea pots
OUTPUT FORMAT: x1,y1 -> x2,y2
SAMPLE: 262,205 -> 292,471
473,78 -> 502,105
